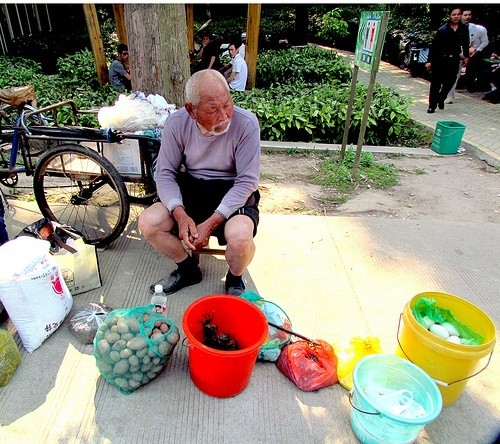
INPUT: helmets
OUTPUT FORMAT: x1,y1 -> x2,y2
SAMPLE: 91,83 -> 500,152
240,33 -> 246,42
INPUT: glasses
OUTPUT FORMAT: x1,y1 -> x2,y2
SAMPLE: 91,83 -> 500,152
122,52 -> 128,56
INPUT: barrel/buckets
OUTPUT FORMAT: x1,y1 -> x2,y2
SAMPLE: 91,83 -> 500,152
181,293 -> 269,397
395,291 -> 496,405
347,353 -> 443,444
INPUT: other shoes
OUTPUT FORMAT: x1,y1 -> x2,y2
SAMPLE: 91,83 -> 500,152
149,266 -> 203,296
445,97 -> 454,104
224,281 -> 247,297
438,101 -> 444,109
427,108 -> 435,113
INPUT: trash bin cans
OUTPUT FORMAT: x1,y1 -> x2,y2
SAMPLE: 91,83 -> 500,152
431,120 -> 467,155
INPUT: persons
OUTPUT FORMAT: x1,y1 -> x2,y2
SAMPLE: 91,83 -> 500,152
109,33 -> 247,94
426,7 -> 489,113
137,69 -> 260,297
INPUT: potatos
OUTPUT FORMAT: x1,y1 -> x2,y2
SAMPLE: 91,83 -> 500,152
93,311 -> 181,391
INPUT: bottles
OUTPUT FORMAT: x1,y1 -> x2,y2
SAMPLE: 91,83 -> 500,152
150,284 -> 167,318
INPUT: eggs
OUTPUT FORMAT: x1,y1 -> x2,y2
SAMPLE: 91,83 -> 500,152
422,316 -> 461,345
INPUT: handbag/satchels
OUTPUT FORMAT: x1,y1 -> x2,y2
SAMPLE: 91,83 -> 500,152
14,217 -> 67,244
48,227 -> 103,296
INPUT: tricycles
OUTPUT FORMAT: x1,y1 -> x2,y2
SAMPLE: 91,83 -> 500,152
0,83 -> 160,249
